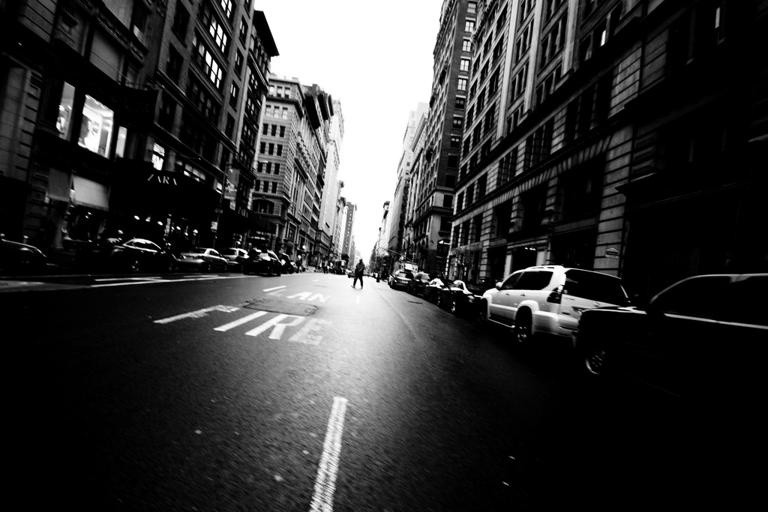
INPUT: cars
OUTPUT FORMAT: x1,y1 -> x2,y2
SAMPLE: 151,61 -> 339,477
387,268 -> 487,318
0,232 -> 46,270
113,236 -> 306,276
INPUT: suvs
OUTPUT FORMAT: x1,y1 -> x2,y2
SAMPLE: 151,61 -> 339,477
477,264 -> 635,359
577,272 -> 768,401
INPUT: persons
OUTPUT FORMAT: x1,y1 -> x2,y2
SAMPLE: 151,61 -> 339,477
296,253 -> 347,275
352,258 -> 364,289
